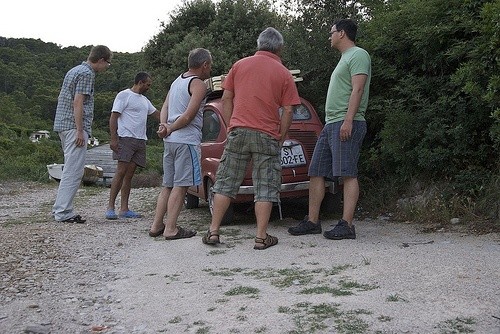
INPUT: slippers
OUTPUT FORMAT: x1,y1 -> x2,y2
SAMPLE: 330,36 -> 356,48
104,209 -> 117,219
119,210 -> 143,217
166,227 -> 198,239
149,224 -> 165,236
62,214 -> 86,223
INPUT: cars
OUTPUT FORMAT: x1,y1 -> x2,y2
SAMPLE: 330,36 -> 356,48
181,93 -> 339,225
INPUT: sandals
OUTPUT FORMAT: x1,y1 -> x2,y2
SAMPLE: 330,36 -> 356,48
254,233 -> 279,250
202,230 -> 219,244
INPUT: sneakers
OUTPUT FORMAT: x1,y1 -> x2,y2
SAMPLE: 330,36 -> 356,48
324,219 -> 356,239
288,215 -> 322,235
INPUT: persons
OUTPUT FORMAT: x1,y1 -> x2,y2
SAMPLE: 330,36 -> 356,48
203,27 -> 301,249
106,73 -> 160,218
150,48 -> 212,239
53,45 -> 112,222
288,20 -> 371,240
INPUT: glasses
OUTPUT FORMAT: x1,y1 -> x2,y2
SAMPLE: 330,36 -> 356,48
103,59 -> 112,66
328,29 -> 342,38
